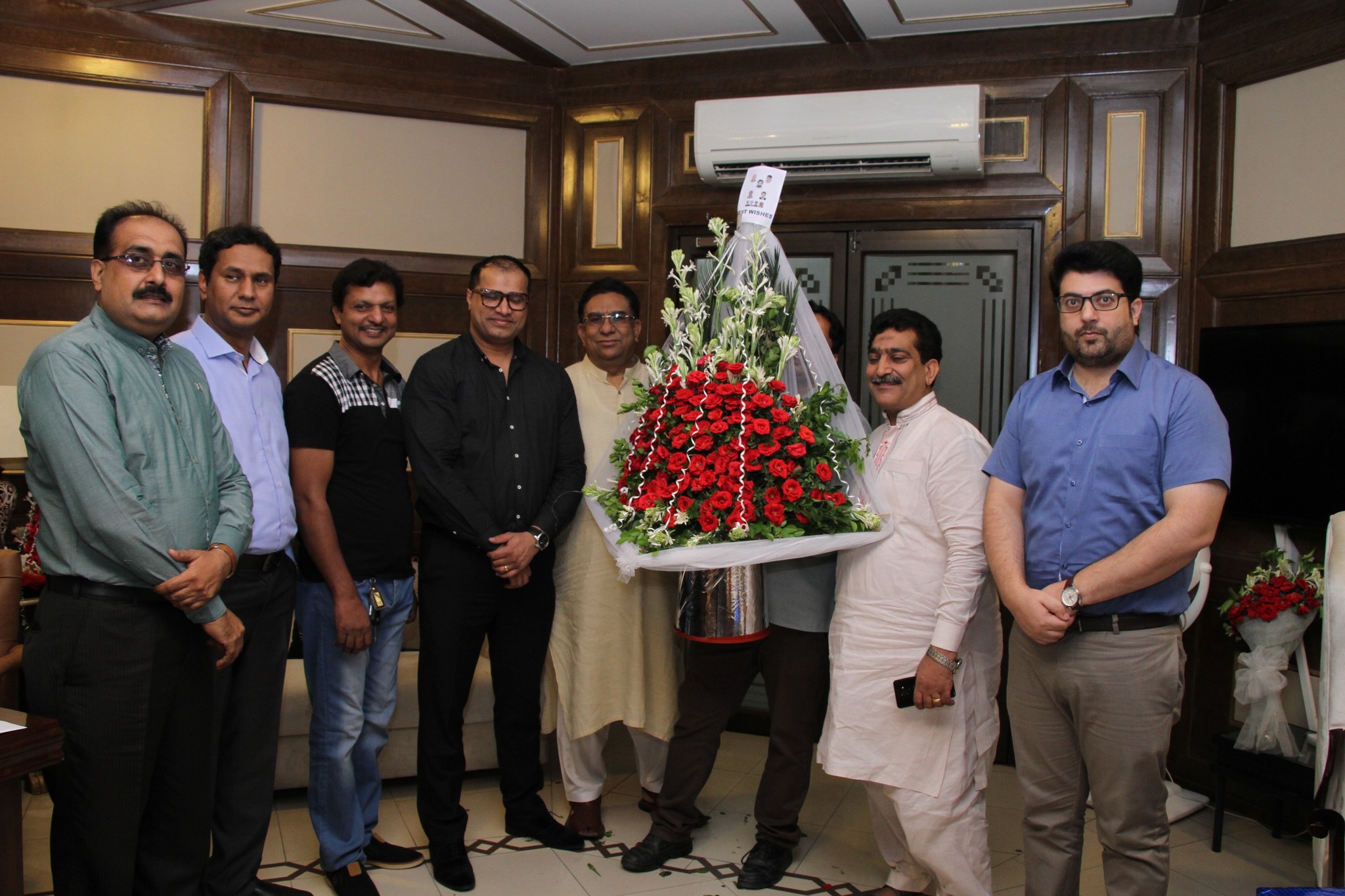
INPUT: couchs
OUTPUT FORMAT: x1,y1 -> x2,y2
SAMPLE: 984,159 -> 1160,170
275,612 -> 554,788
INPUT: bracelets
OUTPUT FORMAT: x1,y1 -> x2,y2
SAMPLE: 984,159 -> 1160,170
206,543 -> 237,578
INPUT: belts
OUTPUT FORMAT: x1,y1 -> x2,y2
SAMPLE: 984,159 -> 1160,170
237,549 -> 288,573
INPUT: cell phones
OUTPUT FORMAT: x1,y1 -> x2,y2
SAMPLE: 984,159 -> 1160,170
893,676 -> 956,708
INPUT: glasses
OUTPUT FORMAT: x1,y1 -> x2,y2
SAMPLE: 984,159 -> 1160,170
472,288 -> 529,311
582,313 -> 635,326
106,252 -> 190,275
1054,291 -> 1131,314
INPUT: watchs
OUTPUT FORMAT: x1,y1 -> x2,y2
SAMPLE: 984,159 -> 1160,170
927,647 -> 963,674
526,527 -> 549,551
1061,576 -> 1087,613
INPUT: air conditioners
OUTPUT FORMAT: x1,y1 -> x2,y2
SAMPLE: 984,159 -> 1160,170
693,83 -> 984,187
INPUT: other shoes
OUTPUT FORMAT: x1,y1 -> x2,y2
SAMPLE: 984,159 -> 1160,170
324,861 -> 380,896
363,832 -> 425,870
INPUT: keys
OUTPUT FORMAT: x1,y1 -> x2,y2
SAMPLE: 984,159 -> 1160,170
371,614 -> 381,642
369,587 -> 377,616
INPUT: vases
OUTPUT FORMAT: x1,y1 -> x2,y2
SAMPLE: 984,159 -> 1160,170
674,562 -> 768,643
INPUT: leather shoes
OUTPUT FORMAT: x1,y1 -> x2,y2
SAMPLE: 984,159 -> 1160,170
505,807 -> 584,853
43,574 -> 175,607
621,833 -> 693,873
428,840 -> 476,892
235,879 -> 315,896
736,842 -> 793,890
638,786 -> 660,813
1066,613 -> 1180,632
564,793 -> 605,841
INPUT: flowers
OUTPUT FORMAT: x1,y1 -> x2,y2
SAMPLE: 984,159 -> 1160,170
581,216 -> 876,555
1217,542 -> 1323,637
11,492 -> 46,576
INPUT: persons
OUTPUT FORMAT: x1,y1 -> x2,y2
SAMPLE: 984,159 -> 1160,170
17,202 -> 254,896
166,221 -> 313,896
982,240 -> 1232,896
403,255 -> 585,892
539,278 -> 710,838
621,300 -> 846,890
280,256 -> 427,896
817,309 -> 1005,896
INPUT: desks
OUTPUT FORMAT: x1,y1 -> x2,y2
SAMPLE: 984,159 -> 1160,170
1214,720 -> 1345,889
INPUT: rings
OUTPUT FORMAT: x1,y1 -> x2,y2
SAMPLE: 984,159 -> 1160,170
504,566 -> 508,572
932,698 -> 941,702
